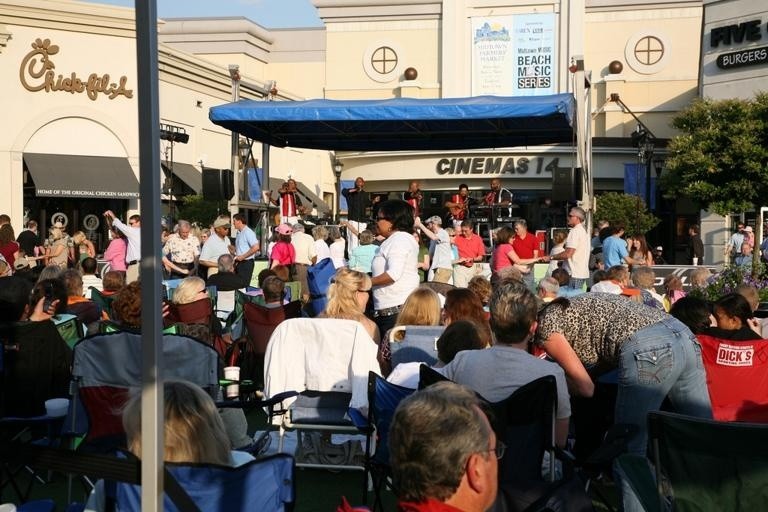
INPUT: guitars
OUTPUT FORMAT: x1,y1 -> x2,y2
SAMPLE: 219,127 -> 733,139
450,191 -> 473,214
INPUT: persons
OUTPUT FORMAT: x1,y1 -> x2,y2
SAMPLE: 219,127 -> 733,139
1,172 -> 767,511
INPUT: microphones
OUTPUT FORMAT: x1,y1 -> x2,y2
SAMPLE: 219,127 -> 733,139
465,191 -> 473,200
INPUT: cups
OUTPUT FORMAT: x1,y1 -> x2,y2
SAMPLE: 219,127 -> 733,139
45,397 -> 69,417
693,258 -> 698,266
224,366 -> 241,398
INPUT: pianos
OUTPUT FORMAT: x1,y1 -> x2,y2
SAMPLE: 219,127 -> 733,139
469,205 -> 520,224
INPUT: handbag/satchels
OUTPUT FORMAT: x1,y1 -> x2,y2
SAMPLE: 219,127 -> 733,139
294,432 -> 363,469
612,455 -> 676,512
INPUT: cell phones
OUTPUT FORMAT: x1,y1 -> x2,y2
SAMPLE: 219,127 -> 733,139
43,283 -> 53,312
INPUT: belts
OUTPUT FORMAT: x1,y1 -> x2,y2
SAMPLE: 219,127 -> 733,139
242,260 -> 253,261
127,261 -> 140,265
375,305 -> 402,316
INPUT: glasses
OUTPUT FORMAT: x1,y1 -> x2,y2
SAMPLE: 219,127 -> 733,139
199,288 -> 208,293
375,216 -> 386,222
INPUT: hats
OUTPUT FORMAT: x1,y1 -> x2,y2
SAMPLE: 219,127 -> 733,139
590,280 -> 641,297
425,216 -> 442,224
656,246 -> 664,252
743,226 -> 752,233
14,257 -> 29,270
51,221 -> 65,230
275,224 -> 293,235
214,218 -> 232,230
